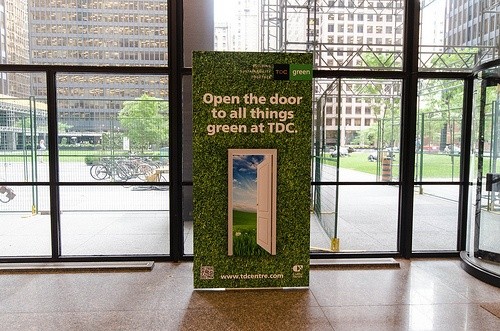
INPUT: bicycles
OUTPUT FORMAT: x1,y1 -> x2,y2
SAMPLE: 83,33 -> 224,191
90,155 -> 166,184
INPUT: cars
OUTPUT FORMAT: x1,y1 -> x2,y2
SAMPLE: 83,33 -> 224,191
368,151 -> 377,161
420,144 -> 460,154
330,149 -> 348,157
